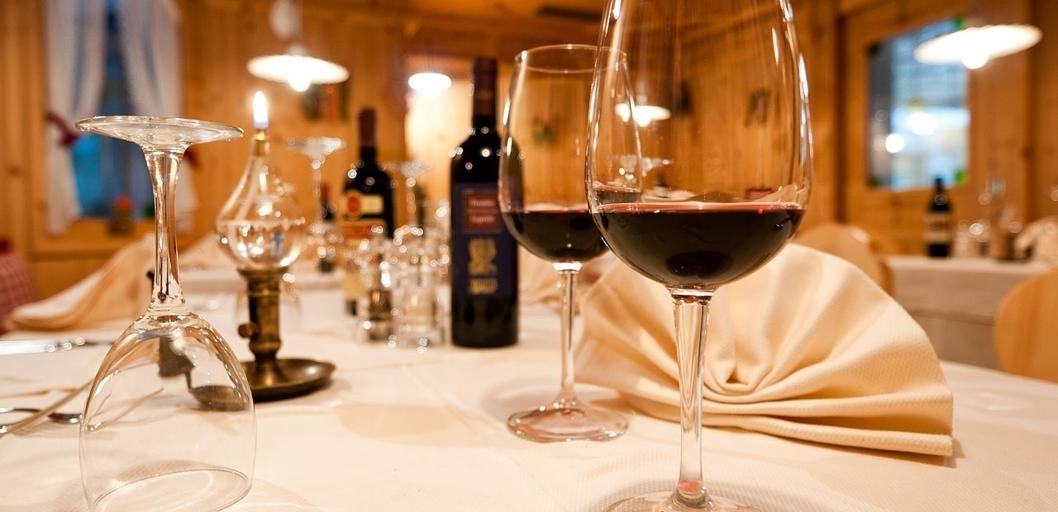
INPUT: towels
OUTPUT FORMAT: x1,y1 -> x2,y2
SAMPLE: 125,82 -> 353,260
573,243 -> 954,457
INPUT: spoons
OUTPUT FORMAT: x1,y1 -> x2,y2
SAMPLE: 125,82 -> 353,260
2,407 -> 83,427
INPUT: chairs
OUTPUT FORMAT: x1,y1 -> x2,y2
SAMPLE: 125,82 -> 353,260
992,263 -> 1058,385
791,222 -> 891,295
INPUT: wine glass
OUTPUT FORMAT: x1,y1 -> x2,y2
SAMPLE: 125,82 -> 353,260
74,115 -> 257,511
286,135 -> 343,284
497,44 -> 641,444
585,0 -> 815,511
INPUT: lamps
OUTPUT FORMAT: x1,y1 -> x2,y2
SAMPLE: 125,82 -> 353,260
914,24 -> 1042,71
633,105 -> 670,127
247,41 -> 348,93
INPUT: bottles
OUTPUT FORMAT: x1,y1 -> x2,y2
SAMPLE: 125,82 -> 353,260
923,177 -> 953,258
449,54 -> 522,350
343,108 -> 395,316
